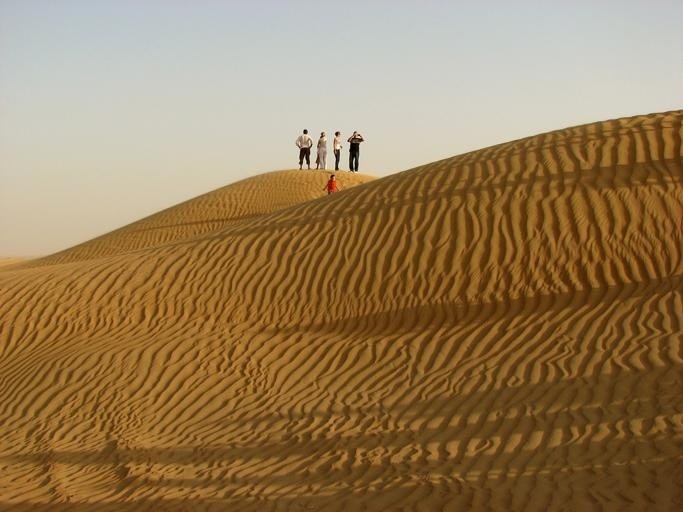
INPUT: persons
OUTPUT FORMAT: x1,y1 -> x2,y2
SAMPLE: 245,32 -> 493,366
318,131 -> 328,170
332,131 -> 342,171
346,131 -> 364,173
314,145 -> 319,170
295,128 -> 313,170
322,175 -> 339,194
